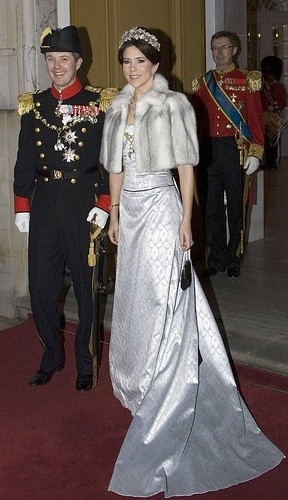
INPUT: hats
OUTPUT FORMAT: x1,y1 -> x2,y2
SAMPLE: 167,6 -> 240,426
39,26 -> 82,54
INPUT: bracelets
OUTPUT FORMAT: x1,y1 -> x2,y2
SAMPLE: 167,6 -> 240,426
109,204 -> 119,209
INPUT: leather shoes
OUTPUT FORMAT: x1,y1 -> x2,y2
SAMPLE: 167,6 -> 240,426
76,373 -> 92,391
30,370 -> 53,387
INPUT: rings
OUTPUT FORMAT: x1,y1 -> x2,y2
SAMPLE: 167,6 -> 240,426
190,241 -> 194,244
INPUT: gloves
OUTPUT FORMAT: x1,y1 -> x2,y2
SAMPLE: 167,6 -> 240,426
13,212 -> 30,232
87,207 -> 109,229
243,157 -> 259,175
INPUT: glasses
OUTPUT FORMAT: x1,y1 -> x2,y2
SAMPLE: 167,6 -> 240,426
211,45 -> 232,49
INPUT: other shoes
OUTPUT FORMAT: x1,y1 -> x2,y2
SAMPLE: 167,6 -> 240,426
227,263 -> 240,277
203,265 -> 225,277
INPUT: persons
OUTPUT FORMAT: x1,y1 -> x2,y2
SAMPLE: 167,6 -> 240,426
258,56 -> 287,170
13,26 -> 120,391
99,27 -> 284,498
193,32 -> 264,278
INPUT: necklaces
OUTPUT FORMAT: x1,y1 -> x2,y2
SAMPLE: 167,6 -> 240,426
128,97 -> 137,111
